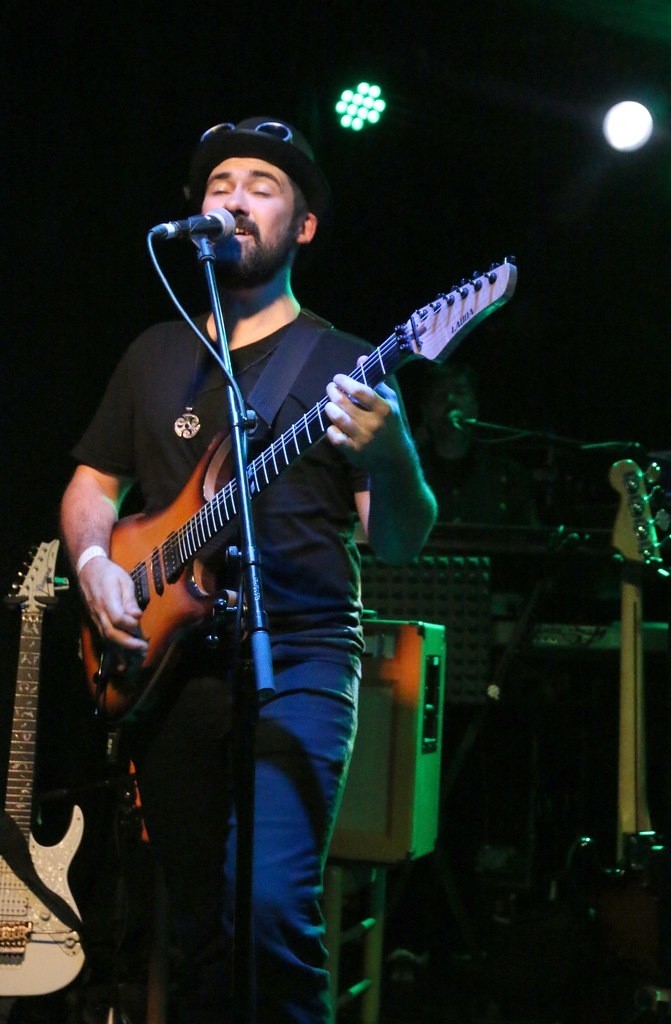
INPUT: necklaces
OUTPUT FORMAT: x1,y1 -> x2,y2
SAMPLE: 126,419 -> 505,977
173,343 -> 279,438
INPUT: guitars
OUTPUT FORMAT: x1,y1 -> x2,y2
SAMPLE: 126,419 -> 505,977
588,452 -> 671,1023
81,250 -> 521,732
0,537 -> 96,1001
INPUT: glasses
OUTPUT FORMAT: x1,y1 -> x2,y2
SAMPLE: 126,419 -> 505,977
200,123 -> 293,143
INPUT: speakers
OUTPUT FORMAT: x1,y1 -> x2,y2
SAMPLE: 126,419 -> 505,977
321,616 -> 448,861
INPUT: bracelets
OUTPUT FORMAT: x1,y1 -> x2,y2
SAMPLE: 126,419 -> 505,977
77,545 -> 107,574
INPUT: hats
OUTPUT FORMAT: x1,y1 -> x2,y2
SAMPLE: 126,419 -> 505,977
189,116 -> 332,218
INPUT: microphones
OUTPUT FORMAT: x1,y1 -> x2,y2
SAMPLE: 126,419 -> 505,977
161,207 -> 238,244
447,408 -> 463,429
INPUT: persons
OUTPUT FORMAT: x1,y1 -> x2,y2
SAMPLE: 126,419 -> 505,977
413,362 -> 545,618
61,118 -> 438,1024
